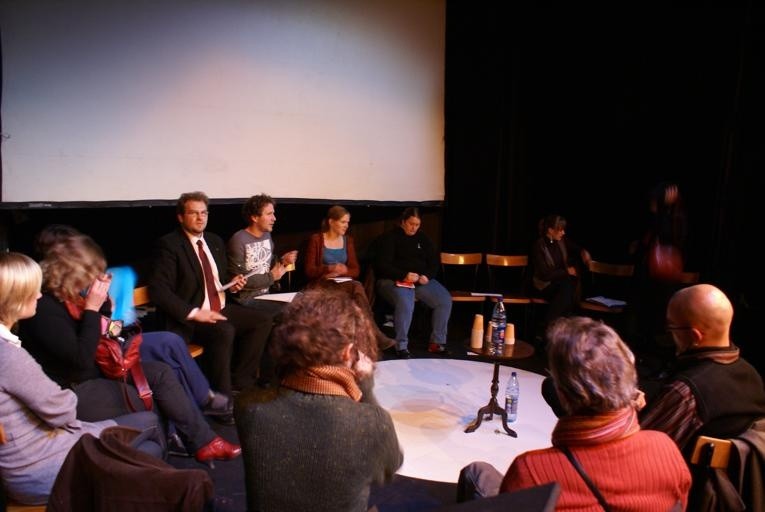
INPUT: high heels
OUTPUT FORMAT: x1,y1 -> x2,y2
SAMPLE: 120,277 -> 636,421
194,434 -> 244,470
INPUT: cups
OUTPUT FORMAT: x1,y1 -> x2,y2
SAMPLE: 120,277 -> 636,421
470,313 -> 484,348
485,320 -> 492,342
505,323 -> 515,345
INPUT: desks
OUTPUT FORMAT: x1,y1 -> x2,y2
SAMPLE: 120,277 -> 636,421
372,358 -> 559,512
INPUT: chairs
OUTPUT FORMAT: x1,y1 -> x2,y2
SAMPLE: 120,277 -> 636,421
485,255 -> 534,336
132,286 -> 203,358
690,435 -> 733,512
442,255 -> 486,313
579,260 -> 634,314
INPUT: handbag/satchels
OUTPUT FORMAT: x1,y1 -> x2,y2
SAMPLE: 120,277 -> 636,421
95,320 -> 143,379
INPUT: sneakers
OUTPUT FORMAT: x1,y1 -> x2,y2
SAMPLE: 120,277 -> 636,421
428,343 -> 449,354
200,393 -> 236,416
398,348 -> 411,360
207,415 -> 236,426
166,433 -> 195,457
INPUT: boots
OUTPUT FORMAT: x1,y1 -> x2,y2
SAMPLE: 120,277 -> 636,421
370,317 -> 397,351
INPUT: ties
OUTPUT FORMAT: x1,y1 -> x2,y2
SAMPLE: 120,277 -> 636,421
196,240 -> 222,315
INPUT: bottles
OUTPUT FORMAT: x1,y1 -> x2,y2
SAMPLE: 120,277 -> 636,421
505,372 -> 521,424
489,296 -> 506,355
